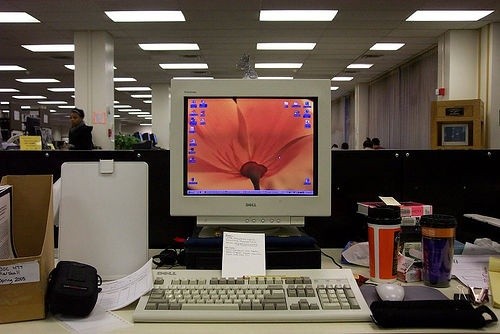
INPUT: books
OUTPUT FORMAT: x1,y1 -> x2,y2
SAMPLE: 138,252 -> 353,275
0,184 -> 19,260
357,201 -> 432,227
488,257 -> 500,308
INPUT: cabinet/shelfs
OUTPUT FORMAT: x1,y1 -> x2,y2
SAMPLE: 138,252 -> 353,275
430,97 -> 485,149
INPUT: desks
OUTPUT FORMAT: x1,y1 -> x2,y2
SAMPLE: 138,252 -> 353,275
0,247 -> 500,333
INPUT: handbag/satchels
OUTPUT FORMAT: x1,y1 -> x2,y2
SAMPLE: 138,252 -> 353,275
45,260 -> 102,316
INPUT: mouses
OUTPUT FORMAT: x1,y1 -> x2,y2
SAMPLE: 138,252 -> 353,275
375,283 -> 406,301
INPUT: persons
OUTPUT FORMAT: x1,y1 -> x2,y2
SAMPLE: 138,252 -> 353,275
66,108 -> 94,149
363,138 -> 384,149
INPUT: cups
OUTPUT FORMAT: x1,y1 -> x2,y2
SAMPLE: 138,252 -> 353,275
366,204 -> 402,284
419,213 -> 458,287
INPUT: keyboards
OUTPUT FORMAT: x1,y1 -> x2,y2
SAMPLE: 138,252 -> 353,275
131,269 -> 373,324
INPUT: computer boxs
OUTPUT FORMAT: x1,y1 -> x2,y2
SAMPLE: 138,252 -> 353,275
184,245 -> 322,269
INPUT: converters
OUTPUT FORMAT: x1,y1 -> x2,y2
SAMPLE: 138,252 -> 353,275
160,249 -> 176,265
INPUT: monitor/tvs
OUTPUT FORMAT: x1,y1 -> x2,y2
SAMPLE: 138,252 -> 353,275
169,78 -> 333,240
